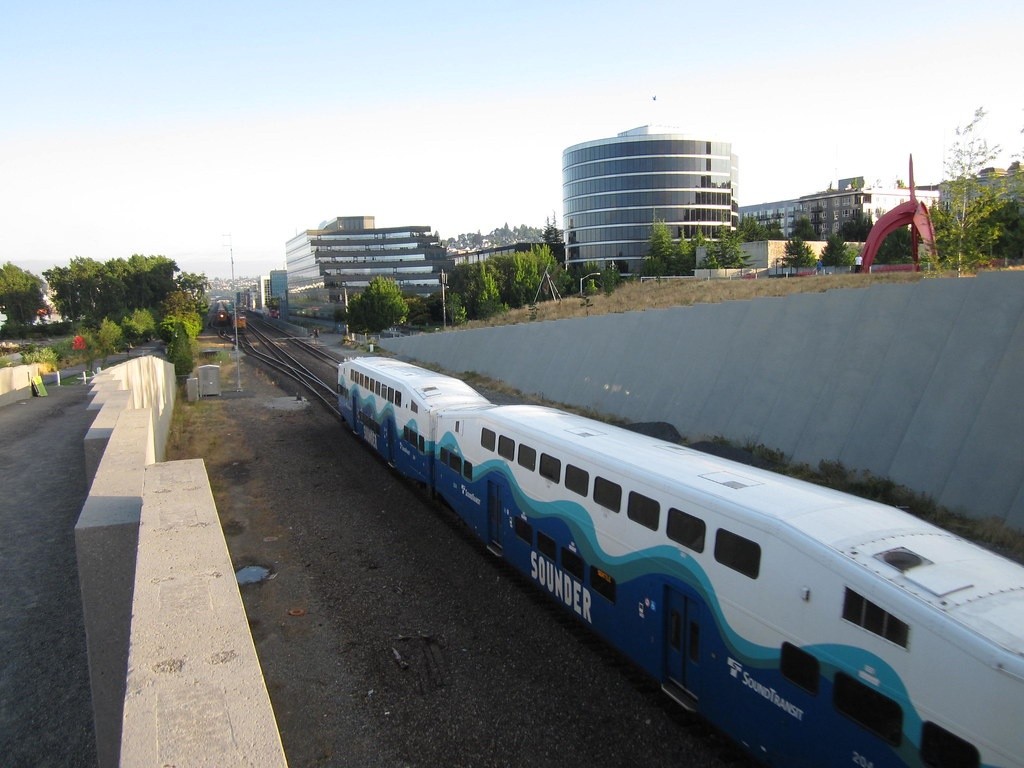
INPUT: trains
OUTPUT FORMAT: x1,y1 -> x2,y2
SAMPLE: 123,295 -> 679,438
215,301 -> 247,330
337,357 -> 1024,767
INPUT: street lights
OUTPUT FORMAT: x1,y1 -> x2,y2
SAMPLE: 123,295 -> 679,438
441,269 -> 450,330
580,272 -> 600,297
